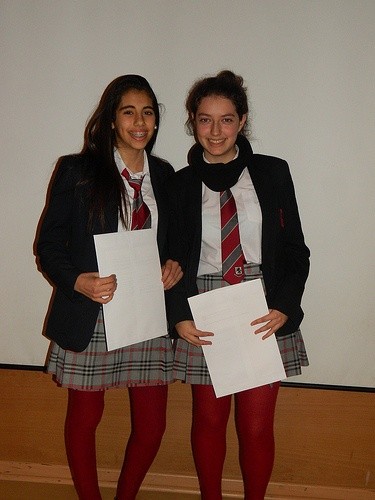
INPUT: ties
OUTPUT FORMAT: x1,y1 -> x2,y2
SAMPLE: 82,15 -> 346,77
219,189 -> 248,285
122,168 -> 151,231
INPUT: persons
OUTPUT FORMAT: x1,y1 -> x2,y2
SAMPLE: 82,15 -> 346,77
33,74 -> 183,500
173,70 -> 310,500
